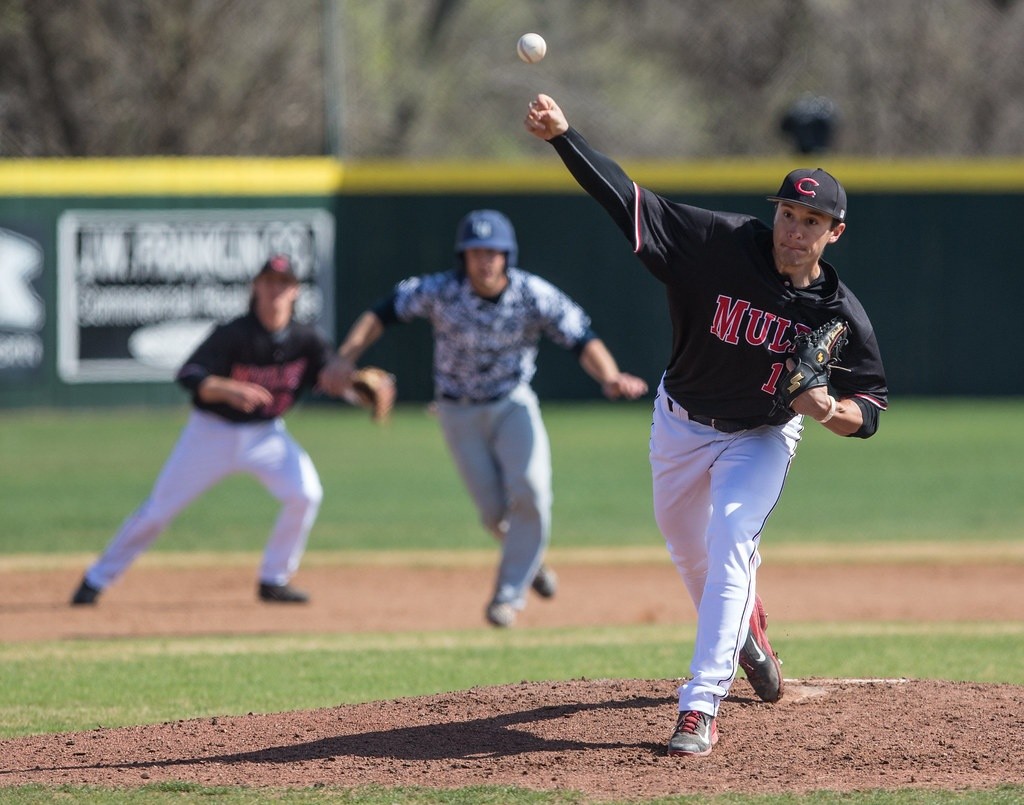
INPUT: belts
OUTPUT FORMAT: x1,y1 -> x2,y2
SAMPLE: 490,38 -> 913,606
665,397 -> 769,433
442,393 -> 502,404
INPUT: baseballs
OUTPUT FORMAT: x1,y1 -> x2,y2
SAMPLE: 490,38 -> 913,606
516,33 -> 547,64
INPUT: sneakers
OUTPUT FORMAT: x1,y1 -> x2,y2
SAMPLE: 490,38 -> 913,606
259,582 -> 310,604
667,709 -> 719,757
737,593 -> 784,703
71,578 -> 98,604
528,564 -> 558,598
487,599 -> 517,628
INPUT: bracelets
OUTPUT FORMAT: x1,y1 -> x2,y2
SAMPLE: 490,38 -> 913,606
819,395 -> 836,424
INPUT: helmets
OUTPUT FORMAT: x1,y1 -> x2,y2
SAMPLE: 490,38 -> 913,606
453,209 -> 518,267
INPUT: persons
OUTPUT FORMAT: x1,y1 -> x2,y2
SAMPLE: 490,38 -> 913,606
72,255 -> 396,605
525,93 -> 889,757
321,208 -> 648,625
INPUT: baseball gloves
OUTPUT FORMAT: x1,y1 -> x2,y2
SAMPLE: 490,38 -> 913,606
350,365 -> 398,425
777,316 -> 855,419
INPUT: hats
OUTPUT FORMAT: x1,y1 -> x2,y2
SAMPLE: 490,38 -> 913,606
252,254 -> 300,283
765,167 -> 847,221
